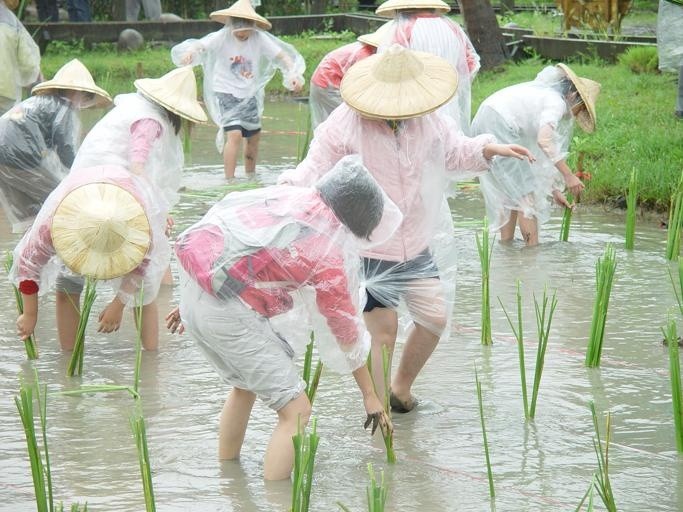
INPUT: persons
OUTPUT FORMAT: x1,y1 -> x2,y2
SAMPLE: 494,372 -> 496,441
170,0 -> 306,179
471,62 -> 602,246
34,0 -> 92,22
164,155 -> 393,480
309,35 -> 378,126
5,156 -> 172,351
1,0 -> 42,121
1,57 -> 112,235
124,1 -> 162,20
374,0 -> 481,197
72,64 -> 207,284
293,41 -> 538,415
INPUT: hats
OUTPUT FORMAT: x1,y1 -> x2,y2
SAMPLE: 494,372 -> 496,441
49,182 -> 153,281
31,58 -> 112,111
373,0 -> 453,19
555,62 -> 602,135
338,43 -> 460,122
209,0 -> 272,31
135,63 -> 209,125
